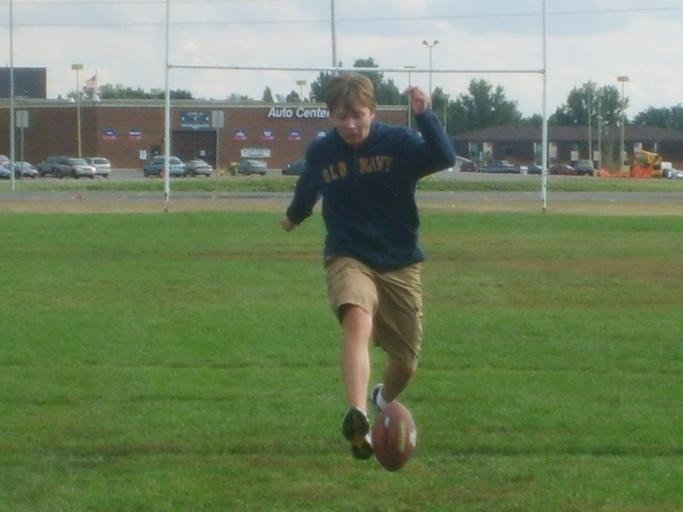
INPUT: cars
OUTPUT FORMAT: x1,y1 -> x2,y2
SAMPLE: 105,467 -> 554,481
55,157 -> 97,179
142,155 -> 187,179
547,163 -> 577,176
663,168 -> 682,180
183,157 -> 213,177
0,154 -> 37,179
228,159 -> 268,176
282,158 -> 306,175
521,164 -> 542,175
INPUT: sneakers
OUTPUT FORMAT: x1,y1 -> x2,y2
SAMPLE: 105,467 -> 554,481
342,384 -> 390,461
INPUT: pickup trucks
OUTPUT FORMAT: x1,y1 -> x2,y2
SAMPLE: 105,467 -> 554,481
478,159 -> 520,174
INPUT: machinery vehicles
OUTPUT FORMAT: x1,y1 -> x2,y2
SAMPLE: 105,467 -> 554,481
630,149 -> 663,178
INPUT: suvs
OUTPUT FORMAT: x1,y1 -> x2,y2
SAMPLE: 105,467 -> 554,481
36,156 -> 72,176
458,162 -> 477,172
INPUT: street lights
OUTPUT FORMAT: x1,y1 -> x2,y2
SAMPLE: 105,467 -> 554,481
616,75 -> 630,172
442,89 -> 451,132
71,63 -> 84,158
421,40 -> 441,111
404,65 -> 417,129
296,79 -> 307,102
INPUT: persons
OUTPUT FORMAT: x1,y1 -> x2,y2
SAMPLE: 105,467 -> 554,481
281,71 -> 458,460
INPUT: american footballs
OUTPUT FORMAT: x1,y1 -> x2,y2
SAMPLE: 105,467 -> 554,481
372,400 -> 416,472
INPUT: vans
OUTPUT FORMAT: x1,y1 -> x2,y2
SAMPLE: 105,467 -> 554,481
569,159 -> 594,176
84,157 -> 111,178
